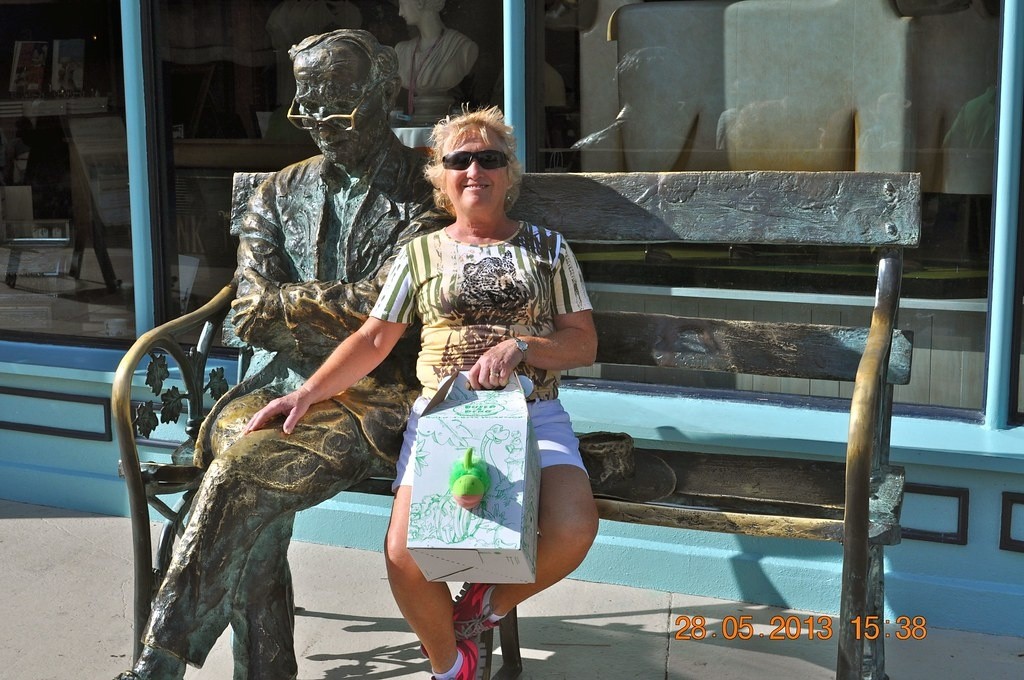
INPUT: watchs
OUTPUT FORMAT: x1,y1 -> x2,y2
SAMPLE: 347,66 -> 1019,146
513,336 -> 529,361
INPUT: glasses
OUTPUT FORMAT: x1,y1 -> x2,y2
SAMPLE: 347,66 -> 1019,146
442,149 -> 510,171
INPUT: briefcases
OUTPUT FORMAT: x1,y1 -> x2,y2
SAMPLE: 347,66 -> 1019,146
406,369 -> 540,584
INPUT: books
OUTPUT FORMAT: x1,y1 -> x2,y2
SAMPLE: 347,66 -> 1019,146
9,39 -> 85,91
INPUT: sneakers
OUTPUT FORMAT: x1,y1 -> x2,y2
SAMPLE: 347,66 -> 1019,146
421,582 -> 502,659
431,640 -> 481,680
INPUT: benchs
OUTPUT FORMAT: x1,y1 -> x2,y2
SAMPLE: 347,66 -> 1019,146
111,172 -> 922,679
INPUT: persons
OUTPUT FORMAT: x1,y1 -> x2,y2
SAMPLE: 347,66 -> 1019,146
395,0 -> 478,90
113,29 -> 453,680
242,115 -> 596,680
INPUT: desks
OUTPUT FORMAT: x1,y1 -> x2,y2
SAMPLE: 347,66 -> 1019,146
0,93 -> 122,299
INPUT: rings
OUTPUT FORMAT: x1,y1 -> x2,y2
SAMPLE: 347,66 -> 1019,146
489,372 -> 500,377
